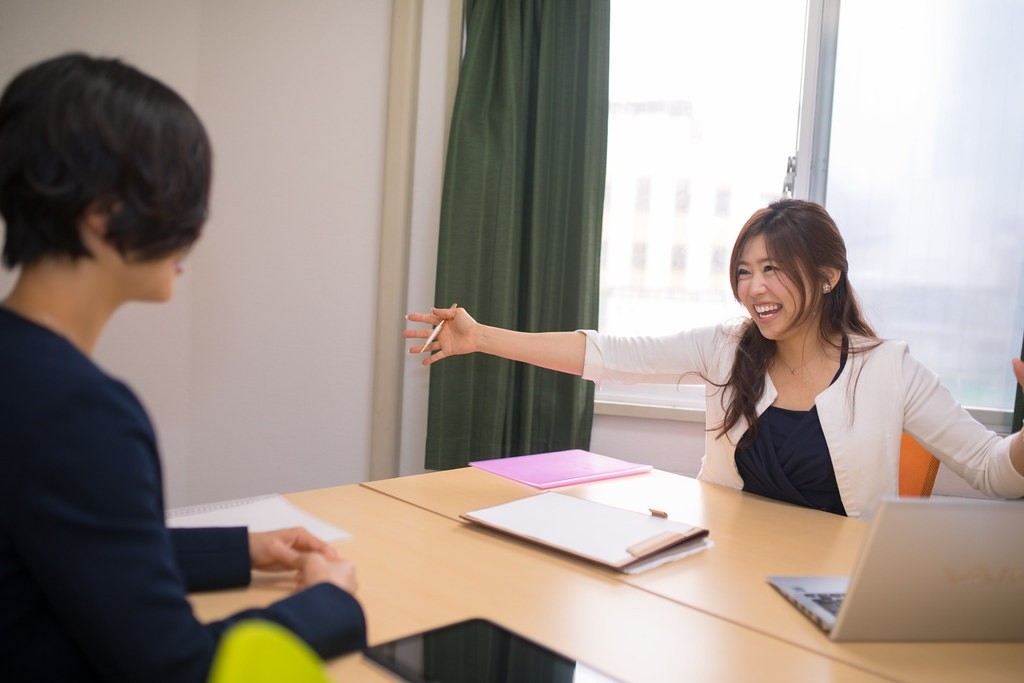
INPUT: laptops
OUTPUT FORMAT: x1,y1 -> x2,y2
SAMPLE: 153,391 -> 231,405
766,497 -> 1024,644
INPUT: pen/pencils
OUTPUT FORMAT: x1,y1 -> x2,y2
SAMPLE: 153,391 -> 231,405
419,303 -> 457,353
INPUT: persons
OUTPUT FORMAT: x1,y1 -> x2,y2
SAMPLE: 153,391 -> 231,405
0,54 -> 366,683
401,200 -> 1024,498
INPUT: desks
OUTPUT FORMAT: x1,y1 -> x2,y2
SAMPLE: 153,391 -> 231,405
165,486 -> 906,683
361,448 -> 1024,683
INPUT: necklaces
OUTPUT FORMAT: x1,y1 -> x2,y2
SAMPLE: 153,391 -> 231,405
778,347 -> 819,373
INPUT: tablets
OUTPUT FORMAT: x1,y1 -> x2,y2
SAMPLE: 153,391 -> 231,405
361,618 -> 624,683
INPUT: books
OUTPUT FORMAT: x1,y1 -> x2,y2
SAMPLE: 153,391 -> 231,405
163,493 -> 350,544
458,491 -> 713,575
468,449 -> 652,489
